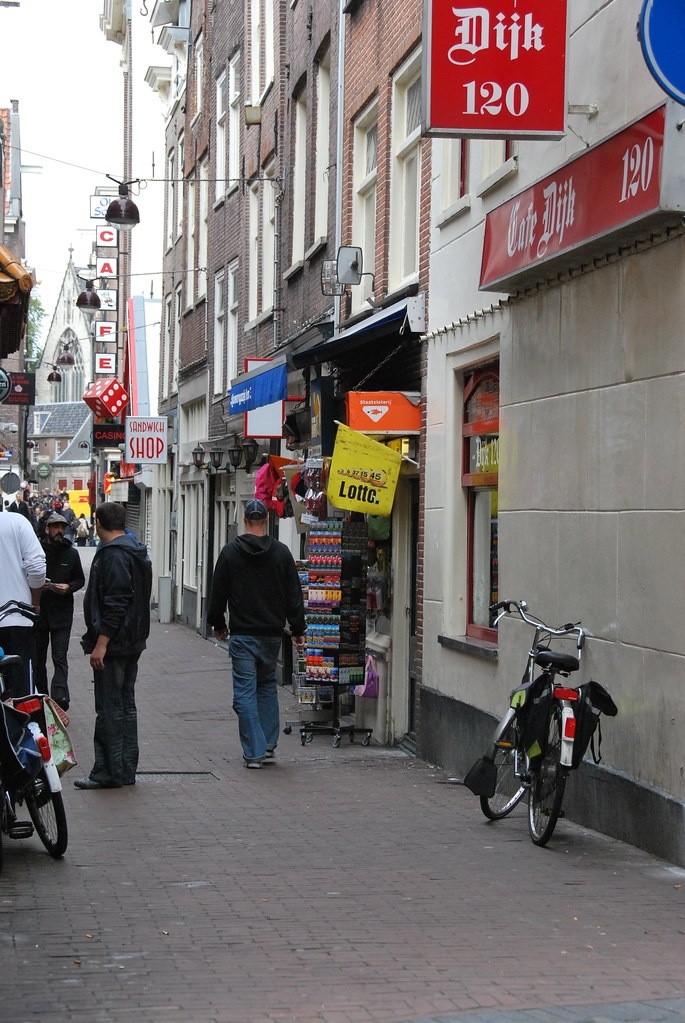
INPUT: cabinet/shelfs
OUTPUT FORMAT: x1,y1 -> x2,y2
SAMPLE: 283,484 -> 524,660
282,518 -> 375,750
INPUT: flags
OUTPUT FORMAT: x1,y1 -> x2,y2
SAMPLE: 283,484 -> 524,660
326,423 -> 403,517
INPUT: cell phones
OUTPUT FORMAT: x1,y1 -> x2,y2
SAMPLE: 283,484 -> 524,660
45,578 -> 51,583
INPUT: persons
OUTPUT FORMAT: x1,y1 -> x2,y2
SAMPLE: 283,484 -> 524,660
29,514 -> 88,714
206,500 -> 308,770
0,509 -> 47,701
0,486 -> 141,546
73,501 -> 152,791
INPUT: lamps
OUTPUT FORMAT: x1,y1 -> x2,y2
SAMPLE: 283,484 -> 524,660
189,441 -> 207,471
103,174 -> 141,233
241,438 -> 259,473
208,442 -> 225,473
45,362 -> 63,386
75,274 -> 102,314
226,439 -> 244,475
56,340 -> 76,370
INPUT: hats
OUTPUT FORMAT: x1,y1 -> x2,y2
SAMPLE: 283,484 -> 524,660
243,498 -> 268,519
46,514 -> 70,526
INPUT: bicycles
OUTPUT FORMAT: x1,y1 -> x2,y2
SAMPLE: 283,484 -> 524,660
466,598 -> 609,845
0,595 -> 71,863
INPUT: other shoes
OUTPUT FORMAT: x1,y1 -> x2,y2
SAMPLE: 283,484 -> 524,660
243,749 -> 277,768
74,777 -> 137,788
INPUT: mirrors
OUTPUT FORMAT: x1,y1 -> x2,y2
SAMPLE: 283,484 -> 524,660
335,245 -> 375,293
320,260 -> 352,321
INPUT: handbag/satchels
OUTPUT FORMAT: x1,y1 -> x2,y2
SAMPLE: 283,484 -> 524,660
0,701 -> 43,809
509,672 -> 551,771
40,693 -> 77,778
567,680 -> 619,771
